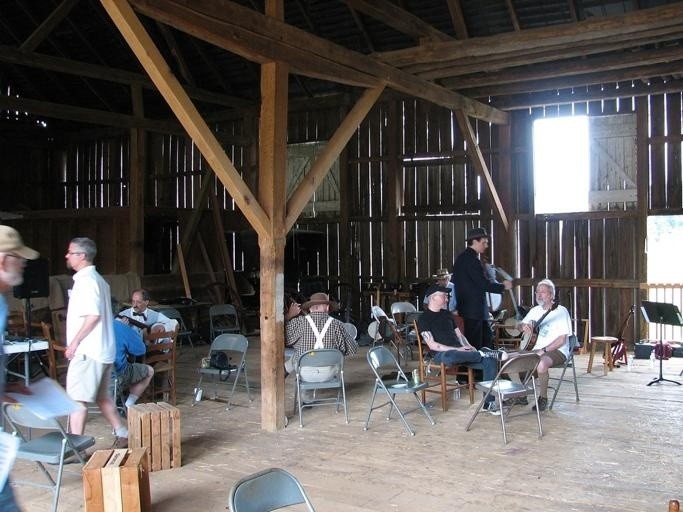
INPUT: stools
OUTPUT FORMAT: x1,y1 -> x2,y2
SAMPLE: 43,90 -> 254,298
586,335 -> 618,376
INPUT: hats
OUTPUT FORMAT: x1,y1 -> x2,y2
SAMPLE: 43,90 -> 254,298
462,226 -> 495,244
431,267 -> 454,281
424,282 -> 454,299
300,289 -> 339,313
0,224 -> 41,261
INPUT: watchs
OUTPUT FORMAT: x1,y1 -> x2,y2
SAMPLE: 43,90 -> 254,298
542,346 -> 546,353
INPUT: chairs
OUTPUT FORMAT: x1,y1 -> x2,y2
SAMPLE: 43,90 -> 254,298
226,465 -> 316,512
192,333 -> 253,410
542,332 -> 580,411
367,300 -> 424,368
412,320 -> 475,413
341,322 -> 357,340
292,346 -> 350,427
43,304 -> 191,414
363,346 -> 436,436
209,303 -> 242,343
466,352 -> 542,444
0,402 -> 96,511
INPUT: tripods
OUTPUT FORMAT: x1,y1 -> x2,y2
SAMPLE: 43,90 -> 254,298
646,324 -> 683,386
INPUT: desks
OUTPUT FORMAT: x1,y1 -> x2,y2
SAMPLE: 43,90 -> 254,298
0,340 -> 49,393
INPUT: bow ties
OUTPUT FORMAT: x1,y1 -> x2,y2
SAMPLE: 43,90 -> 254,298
130,310 -> 146,318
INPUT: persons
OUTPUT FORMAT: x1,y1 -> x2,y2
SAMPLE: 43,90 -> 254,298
109,294 -> 154,419
452,227 -> 512,388
56,236 -> 128,464
420,269 -> 455,313
283,292 -> 358,409
502,279 -> 574,410
416,283 -> 509,415
114,288 -> 178,398
0,221 -> 44,512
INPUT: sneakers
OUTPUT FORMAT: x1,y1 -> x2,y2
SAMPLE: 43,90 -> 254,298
531,395 -> 549,412
48,449 -> 88,466
111,434 -> 129,450
505,394 -> 529,405
480,399 -> 503,417
480,344 -> 509,362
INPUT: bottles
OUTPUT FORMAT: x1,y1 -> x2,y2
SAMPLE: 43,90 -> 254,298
453,381 -> 460,400
412,368 -> 420,384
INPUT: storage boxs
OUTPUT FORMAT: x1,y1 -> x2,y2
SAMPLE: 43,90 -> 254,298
82,447 -> 152,512
126,402 -> 181,472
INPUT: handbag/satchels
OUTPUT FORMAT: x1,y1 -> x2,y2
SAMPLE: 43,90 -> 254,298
206,347 -> 235,382
